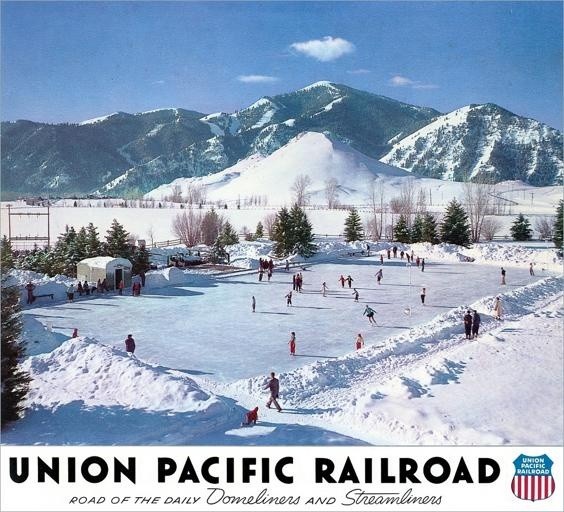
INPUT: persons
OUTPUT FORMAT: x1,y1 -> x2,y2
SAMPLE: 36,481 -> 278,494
71,327 -> 79,339
260,370 -> 282,413
470,309 -> 480,340
353,332 -> 365,351
59,236 -> 550,329
237,405 -> 260,428
123,333 -> 135,353
286,330 -> 296,357
461,309 -> 472,340
23,279 -> 35,306
